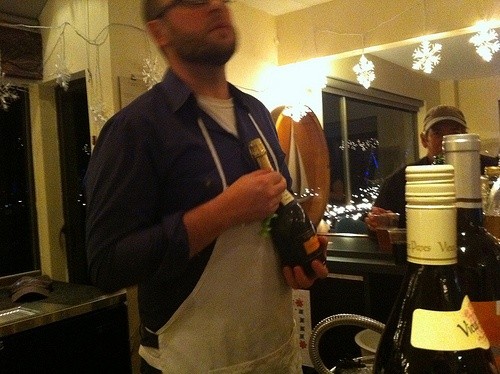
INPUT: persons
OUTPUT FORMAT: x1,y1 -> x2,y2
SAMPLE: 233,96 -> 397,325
364,104 -> 498,264
84,0 -> 329,374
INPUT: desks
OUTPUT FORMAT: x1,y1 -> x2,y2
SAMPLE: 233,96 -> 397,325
1,281 -> 143,374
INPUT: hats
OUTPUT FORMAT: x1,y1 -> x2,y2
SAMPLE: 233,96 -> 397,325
422,104 -> 469,134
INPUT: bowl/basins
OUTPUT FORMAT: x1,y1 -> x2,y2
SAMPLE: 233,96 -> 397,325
355,328 -> 381,353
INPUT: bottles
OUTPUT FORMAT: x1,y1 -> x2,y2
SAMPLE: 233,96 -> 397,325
373,165 -> 499,374
248,138 -> 327,275
443,134 -> 500,374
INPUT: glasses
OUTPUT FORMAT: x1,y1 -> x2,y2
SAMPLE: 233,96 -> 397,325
152,0 -> 209,20
